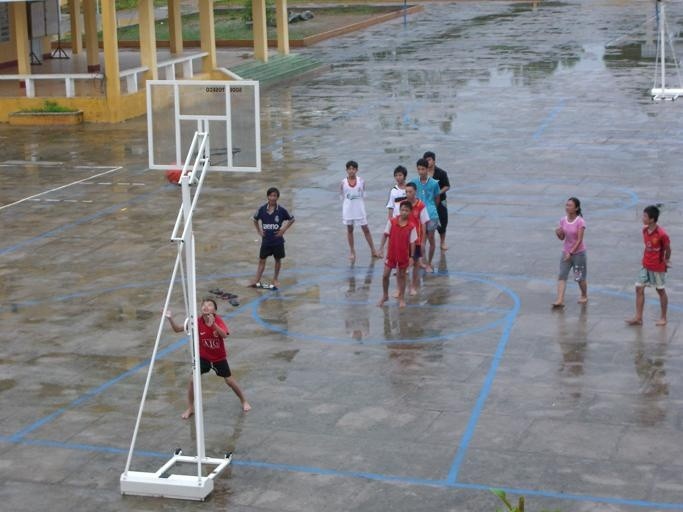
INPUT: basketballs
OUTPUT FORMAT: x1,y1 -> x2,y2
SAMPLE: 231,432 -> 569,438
164,160 -> 191,186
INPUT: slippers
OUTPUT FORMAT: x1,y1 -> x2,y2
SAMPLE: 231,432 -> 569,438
253,282 -> 268,287
263,285 -> 278,290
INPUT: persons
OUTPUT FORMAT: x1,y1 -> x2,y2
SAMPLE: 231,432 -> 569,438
623,203 -> 672,326
245,187 -> 295,288
377,165 -> 407,259
339,160 -> 379,261
392,181 -> 430,298
406,158 -> 441,272
423,151 -> 450,250
379,200 -> 419,310
159,298 -> 252,419
550,196 -> 588,306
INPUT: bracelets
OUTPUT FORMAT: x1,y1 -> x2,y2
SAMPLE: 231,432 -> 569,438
278,230 -> 285,234
